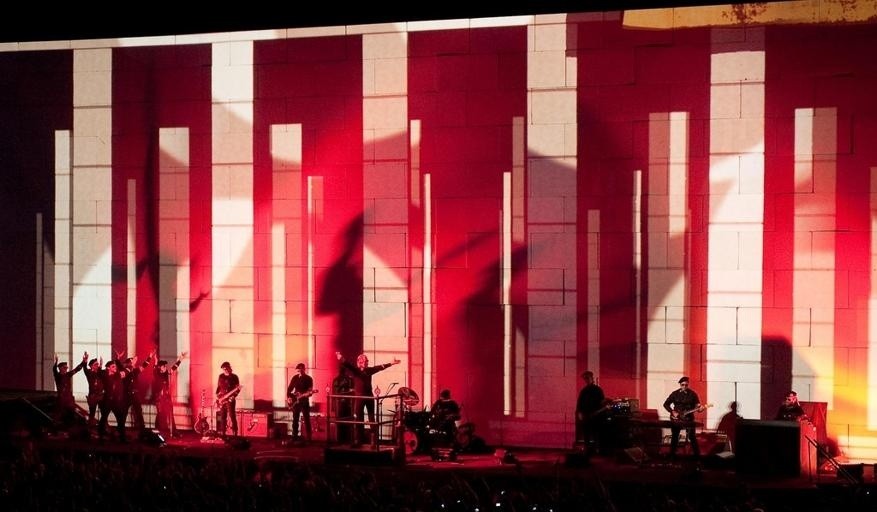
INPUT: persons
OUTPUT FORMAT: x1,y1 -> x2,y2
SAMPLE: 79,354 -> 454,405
53,348 -> 188,439
663,378 -> 704,457
287,363 -> 313,445
330,351 -> 400,448
215,361 -> 240,437
575,372 -> 612,457
421,390 -> 464,463
774,391 -> 808,422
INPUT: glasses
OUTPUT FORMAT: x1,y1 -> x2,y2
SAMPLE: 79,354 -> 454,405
784,398 -> 791,401
681,384 -> 688,386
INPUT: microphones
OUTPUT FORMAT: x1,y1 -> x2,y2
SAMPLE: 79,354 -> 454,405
391,382 -> 399,385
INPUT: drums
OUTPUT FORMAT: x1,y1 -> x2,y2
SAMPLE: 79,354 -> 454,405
395,430 -> 421,454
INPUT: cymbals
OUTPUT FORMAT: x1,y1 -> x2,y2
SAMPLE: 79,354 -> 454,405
398,386 -> 419,406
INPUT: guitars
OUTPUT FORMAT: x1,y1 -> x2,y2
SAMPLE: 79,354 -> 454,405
576,402 -> 610,427
288,390 -> 319,409
670,403 -> 714,426
194,389 -> 210,434
215,384 -> 242,409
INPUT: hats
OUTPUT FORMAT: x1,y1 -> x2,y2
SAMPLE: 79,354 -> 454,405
787,391 -> 796,396
58,362 -> 67,367
221,362 -> 230,368
295,363 -> 305,369
89,357 -> 168,367
679,377 -> 689,383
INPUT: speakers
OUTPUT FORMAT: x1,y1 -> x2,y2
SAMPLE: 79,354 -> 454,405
735,419 -> 801,481
